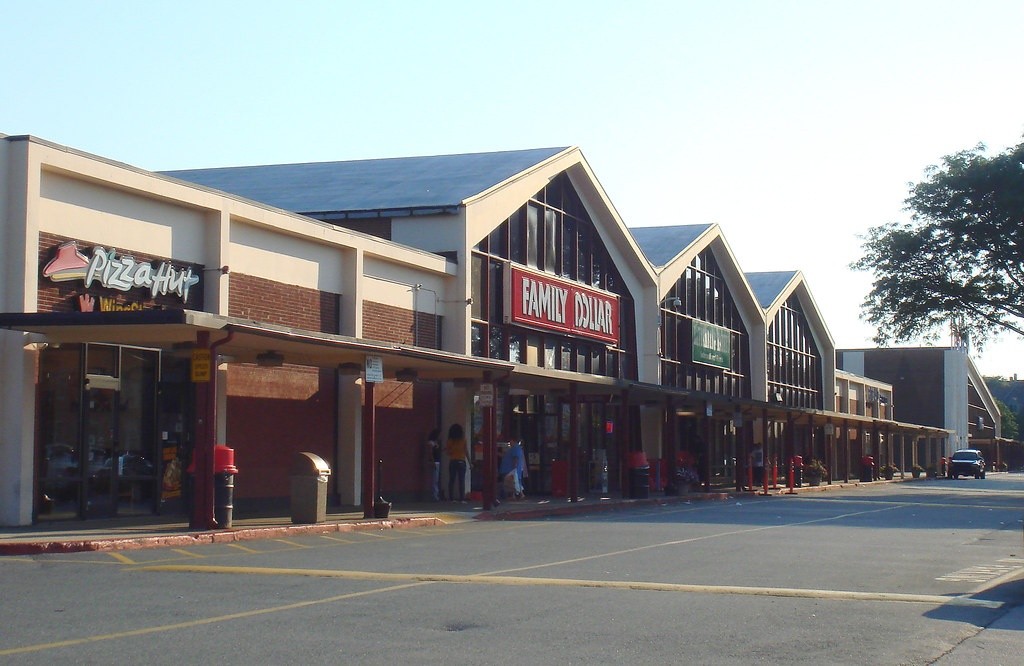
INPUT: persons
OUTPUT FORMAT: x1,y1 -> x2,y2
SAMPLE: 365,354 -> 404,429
446,423 -> 474,504
500,435 -> 528,501
425,427 -> 442,503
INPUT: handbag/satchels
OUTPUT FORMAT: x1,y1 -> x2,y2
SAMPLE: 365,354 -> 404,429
503,467 -> 520,493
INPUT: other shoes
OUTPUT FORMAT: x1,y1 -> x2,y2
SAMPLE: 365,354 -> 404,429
460,500 -> 467,504
448,500 -> 456,504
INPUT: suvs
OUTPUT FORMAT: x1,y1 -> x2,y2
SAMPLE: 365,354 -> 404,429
948,449 -> 985,479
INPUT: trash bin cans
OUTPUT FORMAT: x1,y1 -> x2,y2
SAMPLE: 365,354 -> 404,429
289,452 -> 332,524
187,445 -> 238,528
